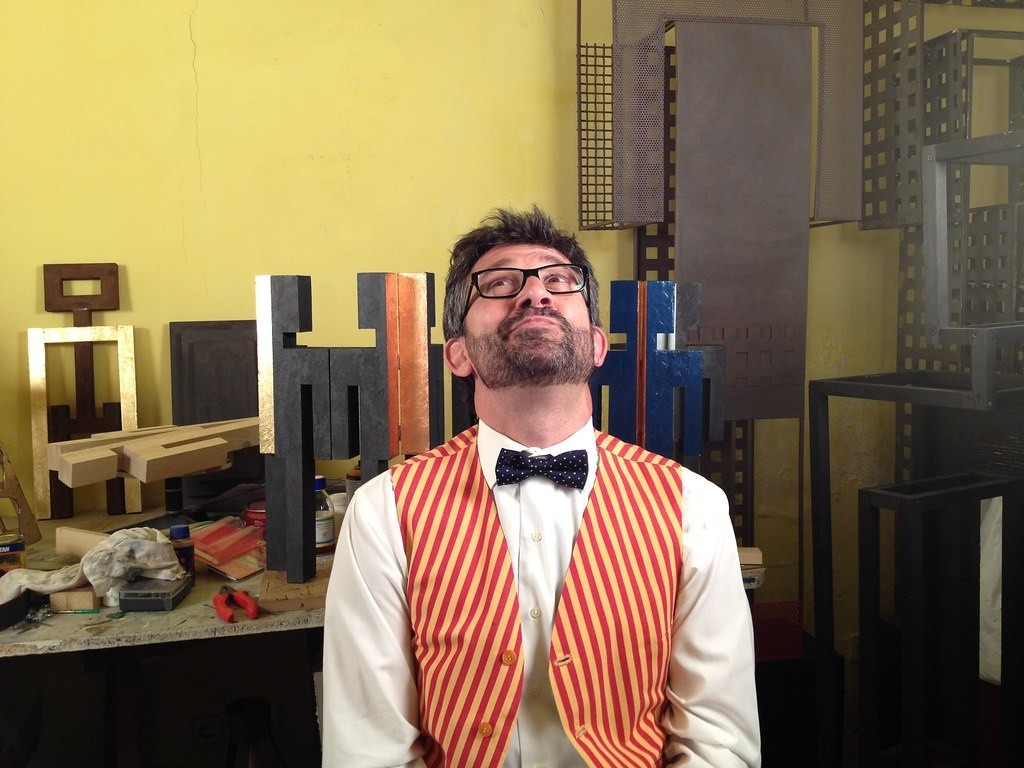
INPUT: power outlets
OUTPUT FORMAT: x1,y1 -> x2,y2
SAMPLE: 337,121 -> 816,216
191,718 -> 224,746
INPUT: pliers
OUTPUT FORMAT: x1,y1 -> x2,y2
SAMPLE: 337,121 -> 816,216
213,584 -> 260,623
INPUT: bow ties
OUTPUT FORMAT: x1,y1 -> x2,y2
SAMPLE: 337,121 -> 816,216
494,447 -> 588,489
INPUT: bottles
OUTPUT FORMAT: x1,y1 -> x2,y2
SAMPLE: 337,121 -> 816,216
169,524 -> 195,586
345,465 -> 362,499
314,475 -> 335,553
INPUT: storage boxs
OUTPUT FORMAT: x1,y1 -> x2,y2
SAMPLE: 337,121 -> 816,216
50,585 -> 102,611
119,573 -> 195,611
0,534 -> 27,578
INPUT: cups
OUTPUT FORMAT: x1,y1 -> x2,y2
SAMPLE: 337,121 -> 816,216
329,491 -> 348,540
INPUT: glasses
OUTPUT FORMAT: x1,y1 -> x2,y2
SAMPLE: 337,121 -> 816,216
457,264 -> 594,329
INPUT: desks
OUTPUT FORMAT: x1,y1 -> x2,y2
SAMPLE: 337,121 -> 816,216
0,512 -> 768,768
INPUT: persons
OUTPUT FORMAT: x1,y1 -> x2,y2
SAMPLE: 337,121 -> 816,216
323,204 -> 763,768
